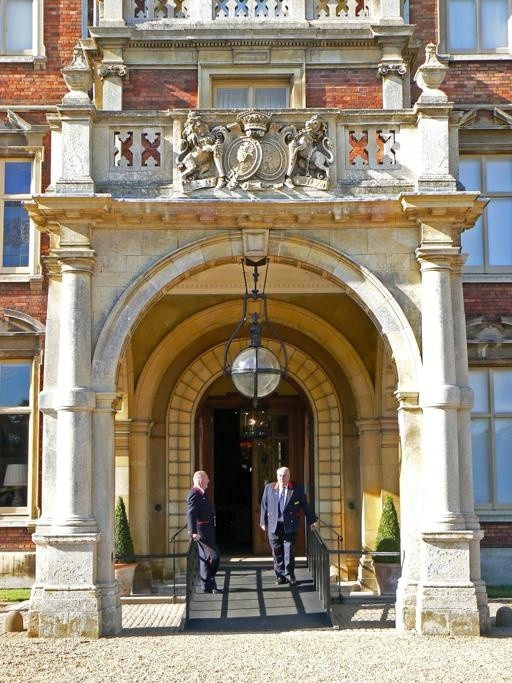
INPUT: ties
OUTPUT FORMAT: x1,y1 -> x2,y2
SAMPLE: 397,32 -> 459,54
278,487 -> 285,518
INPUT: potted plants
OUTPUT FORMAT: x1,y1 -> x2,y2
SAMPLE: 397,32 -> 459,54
370,495 -> 402,596
113,495 -> 138,597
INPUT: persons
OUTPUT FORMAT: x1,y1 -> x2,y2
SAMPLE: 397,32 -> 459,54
259,466 -> 319,585
186,470 -> 225,594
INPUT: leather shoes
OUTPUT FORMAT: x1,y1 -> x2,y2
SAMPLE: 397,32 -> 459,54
275,577 -> 289,585
287,578 -> 297,586
203,584 -> 223,593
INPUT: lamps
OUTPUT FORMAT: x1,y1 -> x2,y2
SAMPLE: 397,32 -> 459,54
222,257 -> 288,408
2,464 -> 28,507
